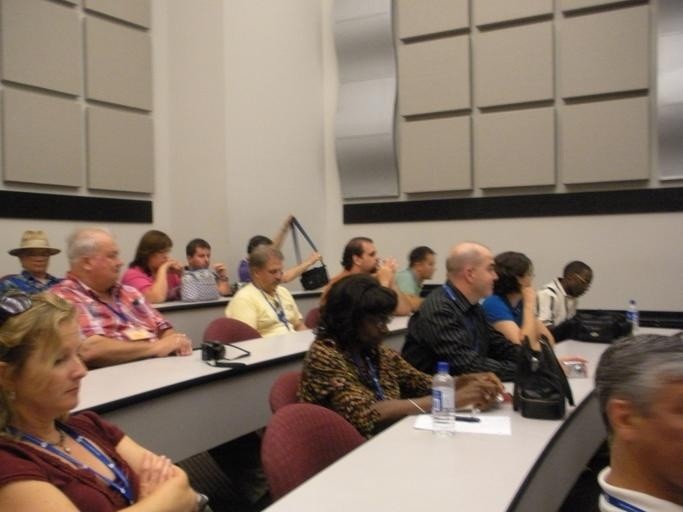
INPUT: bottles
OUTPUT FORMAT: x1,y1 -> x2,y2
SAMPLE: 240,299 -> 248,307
626,297 -> 639,336
431,361 -> 455,427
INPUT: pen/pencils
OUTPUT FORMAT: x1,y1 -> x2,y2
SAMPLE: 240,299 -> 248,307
208,360 -> 246,368
455,417 -> 479,421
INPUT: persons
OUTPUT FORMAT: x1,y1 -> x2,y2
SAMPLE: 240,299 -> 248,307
1,231 -> 555,512
537,261 -> 592,333
596,335 -> 683,512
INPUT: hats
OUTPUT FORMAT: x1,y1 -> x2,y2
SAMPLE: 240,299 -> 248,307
10,231 -> 60,256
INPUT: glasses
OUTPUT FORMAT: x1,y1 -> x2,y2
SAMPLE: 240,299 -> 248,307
0,294 -> 32,325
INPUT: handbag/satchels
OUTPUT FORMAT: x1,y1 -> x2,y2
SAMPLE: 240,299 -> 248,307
180,269 -> 218,302
300,267 -> 328,290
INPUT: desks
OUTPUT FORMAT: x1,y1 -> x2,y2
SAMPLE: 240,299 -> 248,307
62,314 -> 411,471
259,324 -> 680,507
147,287 -> 325,354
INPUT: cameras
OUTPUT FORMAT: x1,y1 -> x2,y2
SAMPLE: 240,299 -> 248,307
202,341 -> 225,361
